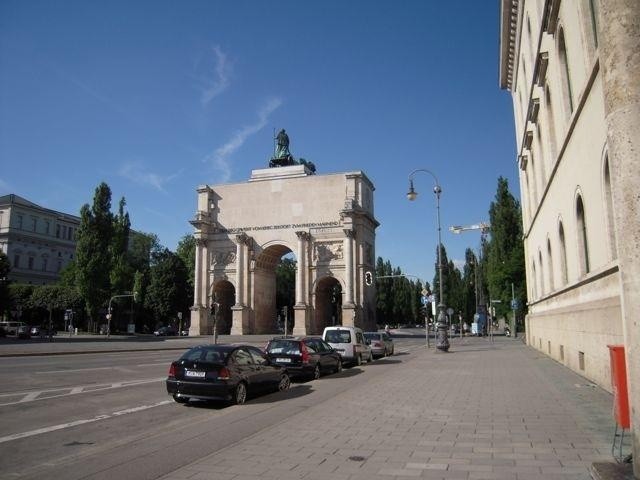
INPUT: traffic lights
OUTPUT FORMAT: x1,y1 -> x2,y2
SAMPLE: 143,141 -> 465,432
210,302 -> 220,317
365,271 -> 372,286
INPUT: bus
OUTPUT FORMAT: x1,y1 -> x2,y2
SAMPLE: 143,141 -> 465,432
0,322 -> 22,335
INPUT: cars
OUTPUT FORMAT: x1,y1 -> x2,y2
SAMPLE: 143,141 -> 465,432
167,326 -> 394,406
154,327 -> 178,337
18,326 -> 56,336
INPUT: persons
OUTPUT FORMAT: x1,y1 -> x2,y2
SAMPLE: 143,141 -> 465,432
273,128 -> 291,159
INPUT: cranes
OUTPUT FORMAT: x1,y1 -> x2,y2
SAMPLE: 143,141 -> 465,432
449,222 -> 491,243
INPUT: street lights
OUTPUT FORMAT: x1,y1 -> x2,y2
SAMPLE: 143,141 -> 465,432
408,169 -> 449,352
